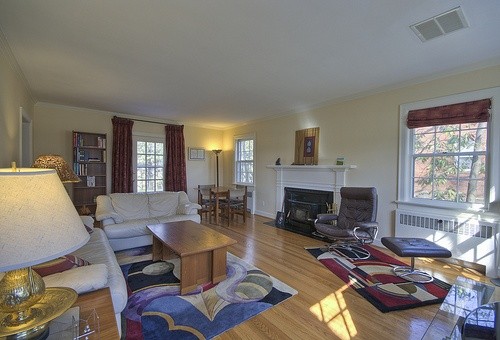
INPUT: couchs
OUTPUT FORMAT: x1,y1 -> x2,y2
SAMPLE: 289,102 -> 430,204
32,214 -> 127,336
94,190 -> 201,252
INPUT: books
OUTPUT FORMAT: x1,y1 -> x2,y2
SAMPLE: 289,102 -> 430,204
92,195 -> 98,203
97,137 -> 106,148
74,133 -> 83,147
75,147 -> 88,162
99,149 -> 106,163
73,162 -> 88,175
88,157 -> 101,162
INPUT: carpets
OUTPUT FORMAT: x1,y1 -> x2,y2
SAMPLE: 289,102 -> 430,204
305,243 -> 453,313
121,251 -> 299,340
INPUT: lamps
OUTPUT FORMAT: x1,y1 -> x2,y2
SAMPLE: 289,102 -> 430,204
0,165 -> 91,340
33,153 -> 82,185
212,148 -> 223,187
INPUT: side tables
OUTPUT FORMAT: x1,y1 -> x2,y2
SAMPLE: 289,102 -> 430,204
48,287 -> 121,340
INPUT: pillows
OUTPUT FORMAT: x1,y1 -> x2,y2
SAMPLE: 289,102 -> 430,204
32,254 -> 90,277
84,222 -> 93,234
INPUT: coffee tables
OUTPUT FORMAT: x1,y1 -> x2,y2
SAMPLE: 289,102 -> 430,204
144,219 -> 239,294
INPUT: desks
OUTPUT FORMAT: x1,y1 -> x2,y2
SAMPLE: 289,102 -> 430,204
380,236 -> 451,285
210,186 -> 240,224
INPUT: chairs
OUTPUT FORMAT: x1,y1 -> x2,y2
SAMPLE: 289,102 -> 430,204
309,186 -> 379,261
221,188 -> 247,226
196,184 -> 220,222
232,184 -> 249,218
200,187 -> 216,224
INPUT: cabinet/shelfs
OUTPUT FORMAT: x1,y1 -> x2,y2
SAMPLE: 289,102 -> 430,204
70,130 -> 108,213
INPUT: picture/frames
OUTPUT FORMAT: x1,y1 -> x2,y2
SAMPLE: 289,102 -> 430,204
188,147 -> 206,161
